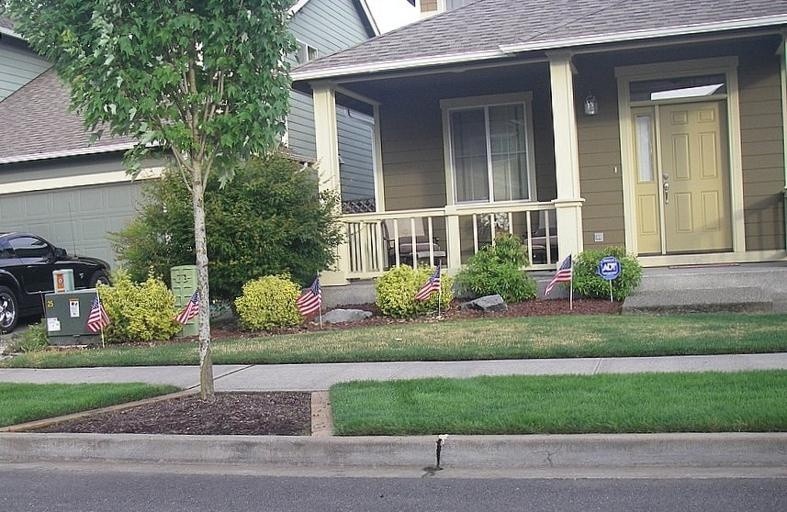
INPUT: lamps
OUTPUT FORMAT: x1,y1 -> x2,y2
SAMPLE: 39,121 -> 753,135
583,90 -> 600,115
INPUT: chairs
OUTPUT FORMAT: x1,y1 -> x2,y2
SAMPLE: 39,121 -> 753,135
380,218 -> 447,269
522,209 -> 558,262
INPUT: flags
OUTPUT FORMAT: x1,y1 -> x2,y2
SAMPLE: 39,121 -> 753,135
296,276 -> 322,316
545,255 -> 572,296
170,287 -> 199,325
87,294 -> 110,334
414,265 -> 441,300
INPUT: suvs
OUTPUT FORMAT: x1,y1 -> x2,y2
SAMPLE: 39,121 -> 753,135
0,231 -> 113,335
